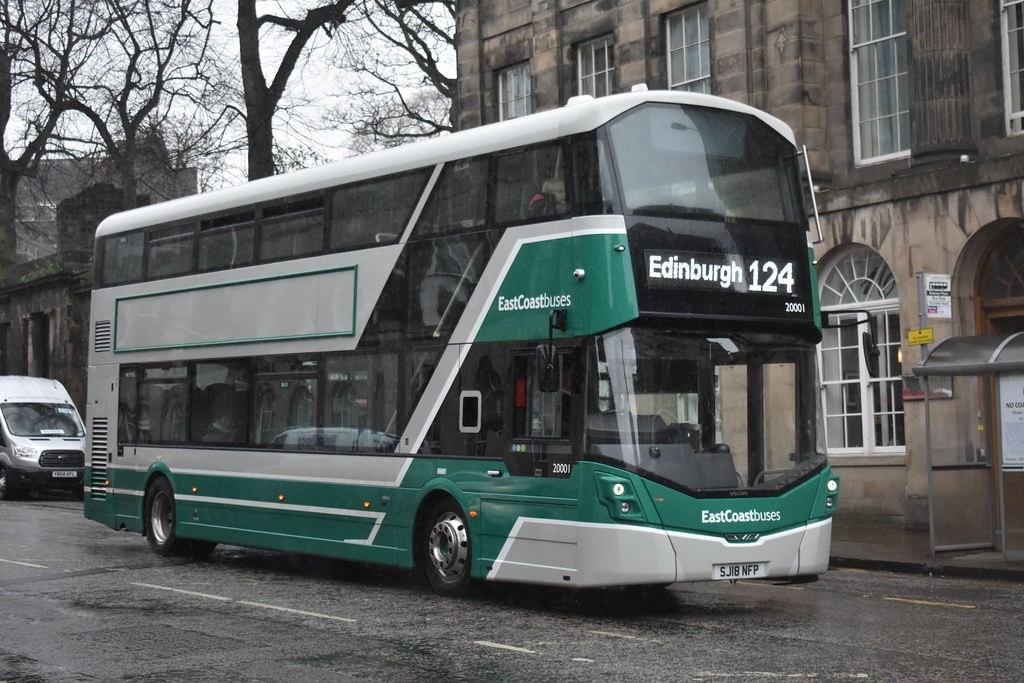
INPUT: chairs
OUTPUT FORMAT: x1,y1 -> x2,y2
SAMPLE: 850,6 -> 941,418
116,375 -> 360,455
112,179 -> 544,286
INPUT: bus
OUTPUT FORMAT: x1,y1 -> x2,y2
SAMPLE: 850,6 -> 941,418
83,82 -> 881,599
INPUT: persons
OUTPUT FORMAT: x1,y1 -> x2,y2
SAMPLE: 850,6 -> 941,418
529,178 -> 566,217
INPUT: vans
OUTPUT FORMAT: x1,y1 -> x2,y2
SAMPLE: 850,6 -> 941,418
0,375 -> 86,501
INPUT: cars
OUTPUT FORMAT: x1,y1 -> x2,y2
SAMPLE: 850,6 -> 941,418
269,428 -> 396,452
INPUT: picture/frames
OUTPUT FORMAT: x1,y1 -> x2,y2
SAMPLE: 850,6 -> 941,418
842,371 -> 862,410
